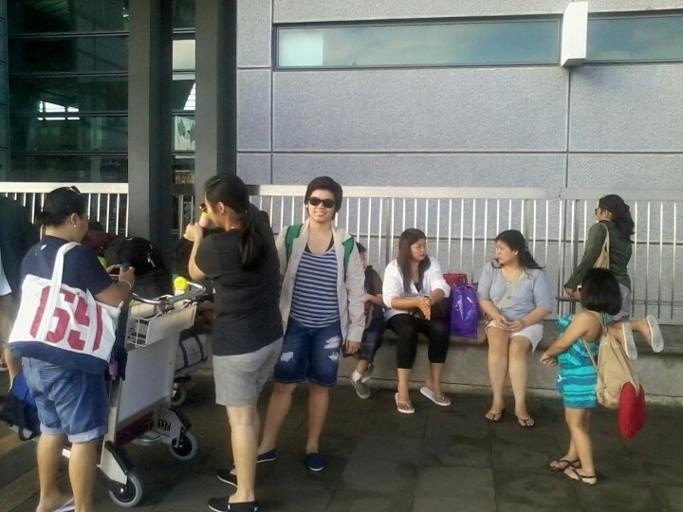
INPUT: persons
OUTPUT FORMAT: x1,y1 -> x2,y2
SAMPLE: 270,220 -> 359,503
234,177 -> 367,473
0,251 -> 21,392
540,267 -> 622,485
564,195 -> 664,362
183,174 -> 285,512
21,186 -> 136,512
476,228 -> 551,427
351,239 -> 383,399
383,229 -> 451,415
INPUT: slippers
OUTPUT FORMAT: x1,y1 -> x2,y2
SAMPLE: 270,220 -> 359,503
394,388 -> 416,417
614,319 -> 638,361
414,382 -> 451,408
637,315 -> 664,353
481,395 -> 505,424
511,411 -> 536,429
35,495 -> 75,512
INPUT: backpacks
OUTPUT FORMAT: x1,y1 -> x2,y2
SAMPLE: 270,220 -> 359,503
595,334 -> 642,410
103,233 -> 173,308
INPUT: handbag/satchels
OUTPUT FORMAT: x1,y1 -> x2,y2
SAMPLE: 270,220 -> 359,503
592,248 -> 613,272
452,281 -> 482,338
5,271 -> 121,376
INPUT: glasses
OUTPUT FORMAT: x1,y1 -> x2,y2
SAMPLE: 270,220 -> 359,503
305,195 -> 338,207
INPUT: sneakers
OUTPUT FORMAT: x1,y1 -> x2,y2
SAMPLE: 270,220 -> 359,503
204,494 -> 260,512
349,373 -> 373,400
254,450 -> 280,463
303,450 -> 325,472
214,466 -> 238,489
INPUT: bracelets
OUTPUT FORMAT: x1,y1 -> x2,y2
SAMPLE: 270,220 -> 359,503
120,278 -> 135,292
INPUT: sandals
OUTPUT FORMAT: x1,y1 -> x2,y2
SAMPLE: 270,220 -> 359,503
547,452 -> 579,472
564,468 -> 596,488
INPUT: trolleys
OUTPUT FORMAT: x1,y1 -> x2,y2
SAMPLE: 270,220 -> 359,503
167,328 -> 206,409
0,263 -> 209,506
